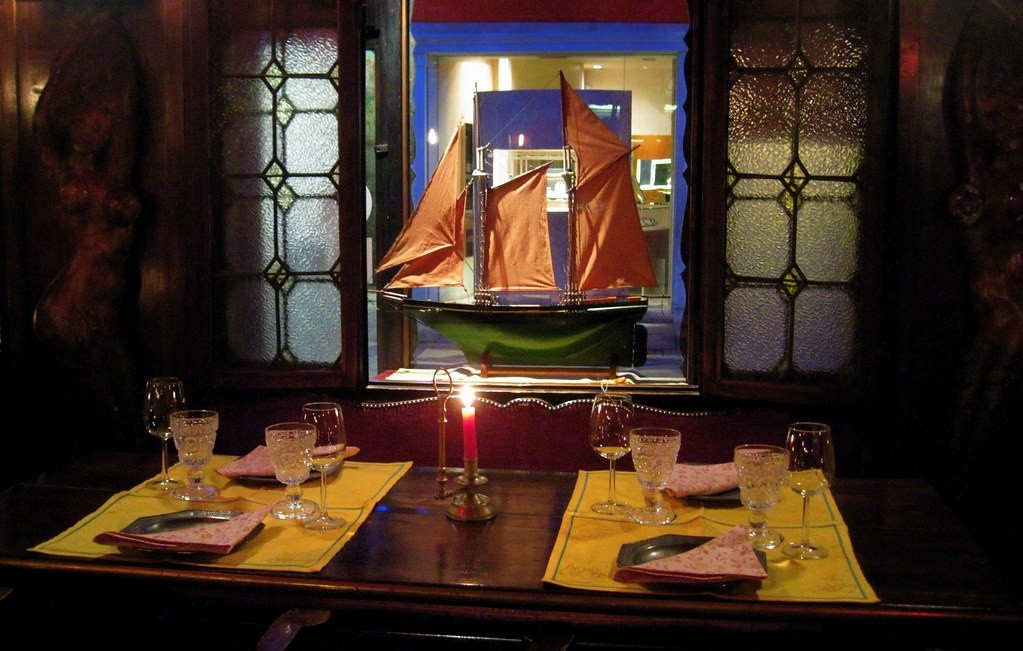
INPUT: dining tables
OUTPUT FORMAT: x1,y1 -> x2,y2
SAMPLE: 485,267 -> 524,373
1,448 -> 1023,651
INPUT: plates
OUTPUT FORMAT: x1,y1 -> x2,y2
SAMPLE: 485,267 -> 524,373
616,534 -> 767,593
238,460 -> 345,481
685,488 -> 743,503
116,509 -> 266,556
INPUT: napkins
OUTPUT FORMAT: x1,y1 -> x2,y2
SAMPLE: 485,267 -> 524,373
90,501 -> 281,554
612,523 -> 767,584
657,463 -> 739,497
212,442 -> 360,479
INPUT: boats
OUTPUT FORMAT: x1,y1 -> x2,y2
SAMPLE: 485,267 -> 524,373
367,67 -> 676,371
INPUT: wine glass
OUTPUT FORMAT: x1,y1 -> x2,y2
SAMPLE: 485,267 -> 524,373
265,422 -> 319,520
143,376 -> 186,490
301,402 -> 347,531
781,421 -> 834,560
170,410 -> 219,501
628,427 -> 682,525
734,444 -> 790,552
587,392 -> 635,516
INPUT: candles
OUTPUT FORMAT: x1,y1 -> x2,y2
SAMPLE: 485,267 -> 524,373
460,385 -> 477,481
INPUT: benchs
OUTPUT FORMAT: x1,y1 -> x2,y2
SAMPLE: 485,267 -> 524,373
320,394 -> 805,471
